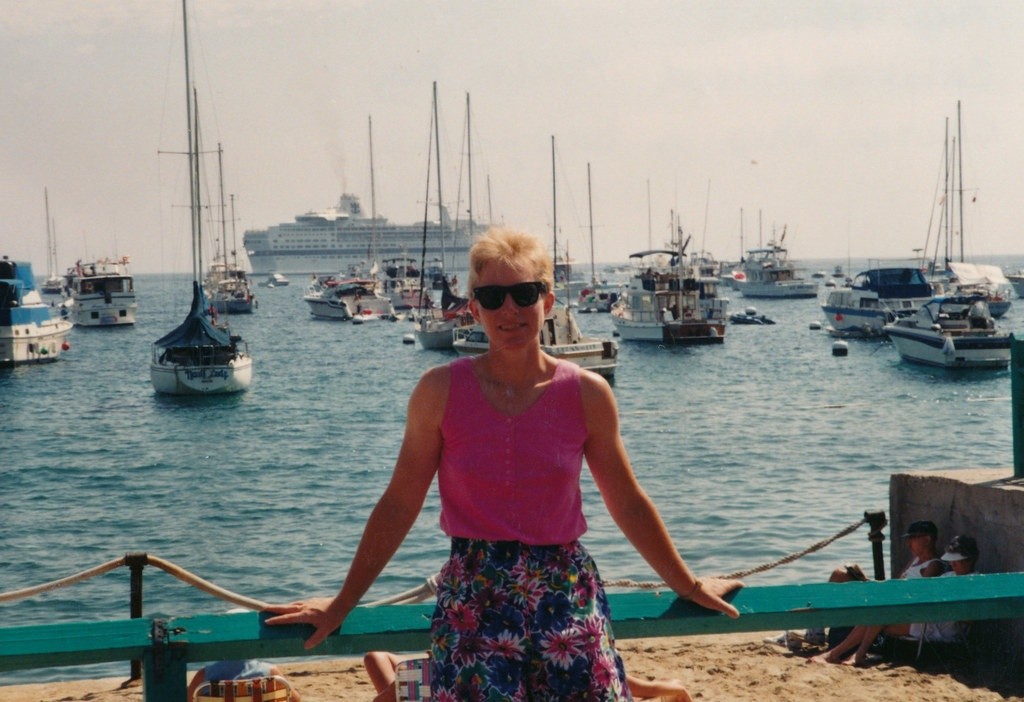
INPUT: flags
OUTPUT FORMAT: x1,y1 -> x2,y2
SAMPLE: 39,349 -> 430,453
835,310 -> 843,321
732,270 -> 750,283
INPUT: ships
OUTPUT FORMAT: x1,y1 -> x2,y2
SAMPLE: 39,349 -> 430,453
241,192 -> 492,276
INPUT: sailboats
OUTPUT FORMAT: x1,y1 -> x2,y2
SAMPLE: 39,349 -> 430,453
364,80 -> 619,384
148,0 -> 261,396
35,187 -> 75,319
819,99 -> 1023,337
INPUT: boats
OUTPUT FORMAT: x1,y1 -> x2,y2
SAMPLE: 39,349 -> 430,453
879,291 -> 1017,369
59,257 -> 138,327
611,180 -> 819,346
0,255 -> 74,365
258,272 -> 291,287
297,276 -> 401,324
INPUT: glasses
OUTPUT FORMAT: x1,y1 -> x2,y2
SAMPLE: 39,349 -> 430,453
472,282 -> 548,311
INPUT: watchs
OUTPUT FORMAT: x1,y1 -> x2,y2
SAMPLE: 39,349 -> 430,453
680,578 -> 703,601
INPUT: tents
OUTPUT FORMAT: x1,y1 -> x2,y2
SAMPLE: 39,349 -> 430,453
187,659 -> 300,702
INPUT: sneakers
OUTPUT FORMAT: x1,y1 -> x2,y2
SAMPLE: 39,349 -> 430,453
763,633 -> 802,650
791,630 -> 826,646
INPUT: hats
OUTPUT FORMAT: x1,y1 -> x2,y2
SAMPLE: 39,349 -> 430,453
902,520 -> 938,539
940,536 -> 979,562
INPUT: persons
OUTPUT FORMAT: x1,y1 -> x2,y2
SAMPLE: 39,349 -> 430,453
803,520 -> 979,667
259,228 -> 747,702
364,651 -> 692,702
